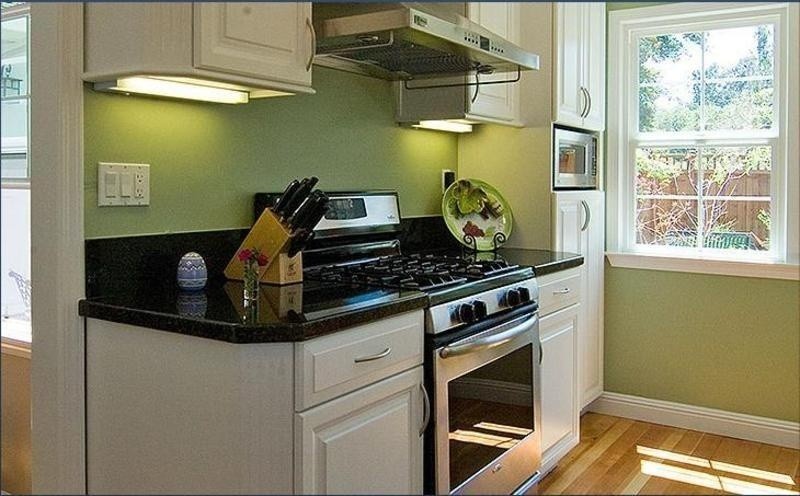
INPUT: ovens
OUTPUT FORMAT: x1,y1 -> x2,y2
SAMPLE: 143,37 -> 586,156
422,299 -> 546,496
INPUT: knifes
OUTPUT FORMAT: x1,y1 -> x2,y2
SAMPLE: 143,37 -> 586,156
273,175 -> 330,235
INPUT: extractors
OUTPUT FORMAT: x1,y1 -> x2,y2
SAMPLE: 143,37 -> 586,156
311,3 -> 537,92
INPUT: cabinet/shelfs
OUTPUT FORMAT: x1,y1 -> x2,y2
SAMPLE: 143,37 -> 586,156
536,264 -> 582,476
394,2 -> 522,132
84,1 -> 318,104
498,191 -> 605,406
521,2 -> 607,130
86,309 -> 429,495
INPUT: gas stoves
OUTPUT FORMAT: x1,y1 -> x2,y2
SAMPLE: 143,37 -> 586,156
302,249 -> 540,339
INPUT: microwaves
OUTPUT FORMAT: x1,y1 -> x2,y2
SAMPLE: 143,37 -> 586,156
553,124 -> 602,193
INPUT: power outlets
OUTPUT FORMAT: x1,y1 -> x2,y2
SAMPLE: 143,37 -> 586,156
98,163 -> 151,207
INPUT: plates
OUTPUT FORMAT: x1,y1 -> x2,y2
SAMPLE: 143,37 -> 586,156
441,178 -> 513,253
